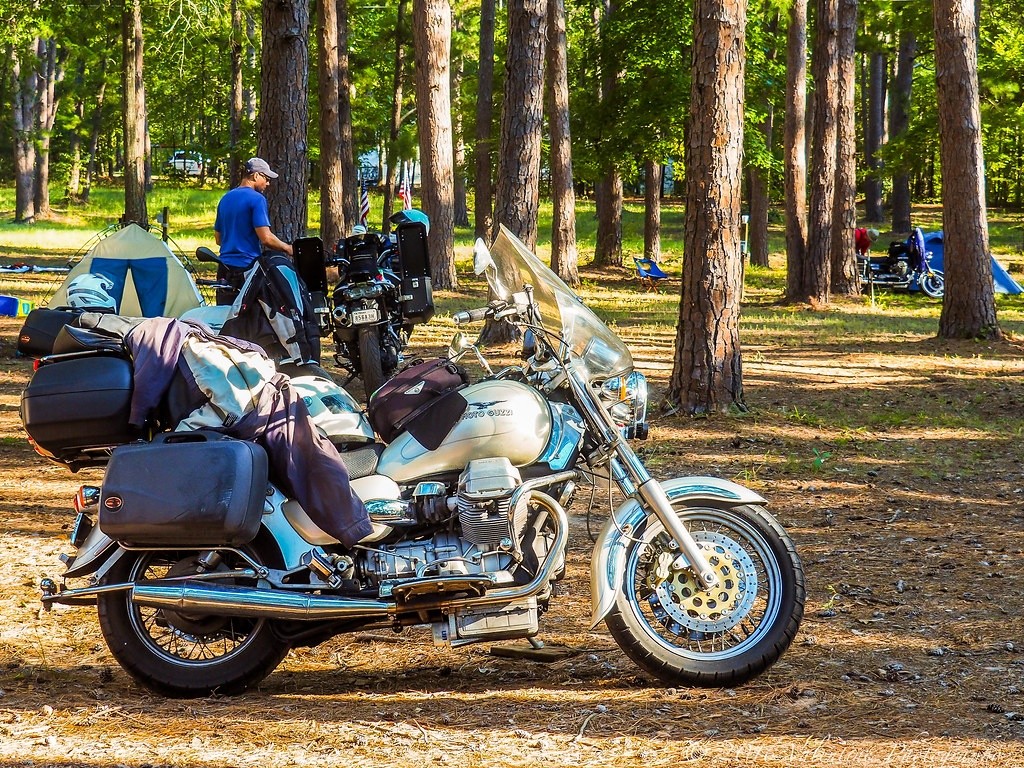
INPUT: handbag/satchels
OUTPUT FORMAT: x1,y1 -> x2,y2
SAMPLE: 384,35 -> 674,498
365,358 -> 470,450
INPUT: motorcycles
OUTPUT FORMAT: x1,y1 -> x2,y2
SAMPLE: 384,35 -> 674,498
177,245 -> 308,360
856,223 -> 944,300
15,221 -> 808,700
302,219 -> 436,404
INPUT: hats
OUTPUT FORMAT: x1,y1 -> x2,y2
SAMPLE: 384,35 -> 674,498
245,157 -> 279,179
867,229 -> 878,243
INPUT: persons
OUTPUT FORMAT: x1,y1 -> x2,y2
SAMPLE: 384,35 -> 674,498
215,158 -> 292,305
855,226 -> 879,255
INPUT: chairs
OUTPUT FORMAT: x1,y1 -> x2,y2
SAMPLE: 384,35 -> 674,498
634,256 -> 667,293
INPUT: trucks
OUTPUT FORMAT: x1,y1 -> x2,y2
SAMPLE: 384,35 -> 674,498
358,146 -> 383,186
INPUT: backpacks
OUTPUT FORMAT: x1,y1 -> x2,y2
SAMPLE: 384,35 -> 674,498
888,241 -> 909,258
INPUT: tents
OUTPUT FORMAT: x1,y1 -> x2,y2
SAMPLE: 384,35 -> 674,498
901,231 -> 1024,294
40,221 -> 212,320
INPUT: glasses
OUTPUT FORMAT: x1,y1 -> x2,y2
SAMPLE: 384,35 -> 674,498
250,172 -> 270,182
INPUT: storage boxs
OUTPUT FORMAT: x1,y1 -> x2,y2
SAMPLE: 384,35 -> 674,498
97,430 -> 269,547
293,236 -> 329,337
21,355 -> 137,456
396,221 -> 435,324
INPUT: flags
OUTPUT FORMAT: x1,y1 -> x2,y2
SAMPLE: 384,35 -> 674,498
360,176 -> 370,230
399,165 -> 412,212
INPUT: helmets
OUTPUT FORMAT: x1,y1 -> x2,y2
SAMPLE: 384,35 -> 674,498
290,375 -> 375,445
66,273 -> 117,314
353,225 -> 366,235
401,209 -> 430,237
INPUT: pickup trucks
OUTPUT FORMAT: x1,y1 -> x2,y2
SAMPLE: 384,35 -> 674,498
168,150 -> 204,177
